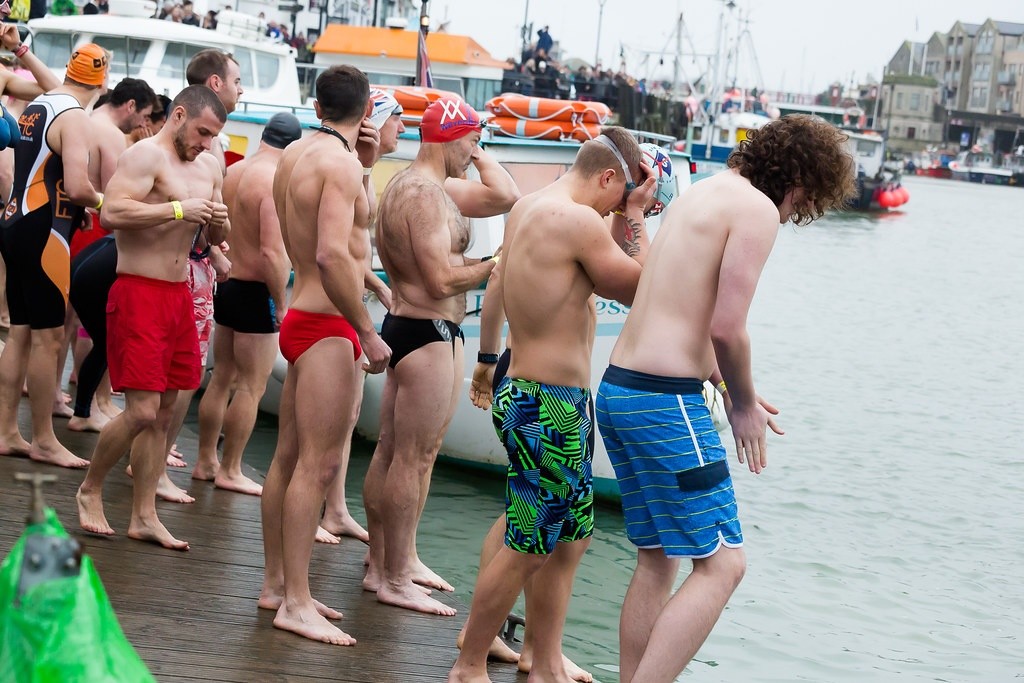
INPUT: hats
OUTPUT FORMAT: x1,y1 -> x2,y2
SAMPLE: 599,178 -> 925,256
365,88 -> 398,129
419,95 -> 483,143
262,113 -> 302,150
639,143 -> 675,208
66,44 -> 106,85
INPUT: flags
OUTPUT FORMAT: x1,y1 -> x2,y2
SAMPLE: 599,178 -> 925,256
419,27 -> 435,89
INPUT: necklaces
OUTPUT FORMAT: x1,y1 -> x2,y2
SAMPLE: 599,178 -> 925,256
312,122 -> 352,153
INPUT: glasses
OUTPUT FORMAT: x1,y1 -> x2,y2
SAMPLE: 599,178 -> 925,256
392,105 -> 404,115
643,201 -> 662,218
621,181 -> 637,205
189,244 -> 211,262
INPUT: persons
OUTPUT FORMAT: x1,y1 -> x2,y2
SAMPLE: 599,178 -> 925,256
250,12 -> 330,78
0,0 -> 243,551
192,112 -> 302,495
596,114 -> 856,683
448,126 -> 674,683
259,66 -> 393,646
502,57 -> 687,139
363,94 -> 522,616
535,24 -> 553,55
315,91 -> 406,544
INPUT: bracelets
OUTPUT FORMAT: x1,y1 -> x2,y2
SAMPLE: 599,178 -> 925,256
477,351 -> 500,365
491,256 -> 500,264
717,380 -> 727,393
481,256 -> 492,262
172,200 -> 184,221
615,210 -> 625,216
15,46 -> 27,58
94,193 -> 104,210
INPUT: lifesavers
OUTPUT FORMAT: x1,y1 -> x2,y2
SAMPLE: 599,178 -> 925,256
482,91 -> 613,142
367,82 -> 464,129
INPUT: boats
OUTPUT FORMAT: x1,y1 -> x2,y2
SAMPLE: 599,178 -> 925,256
0,0 -> 902,513
885,142 -> 1024,186
